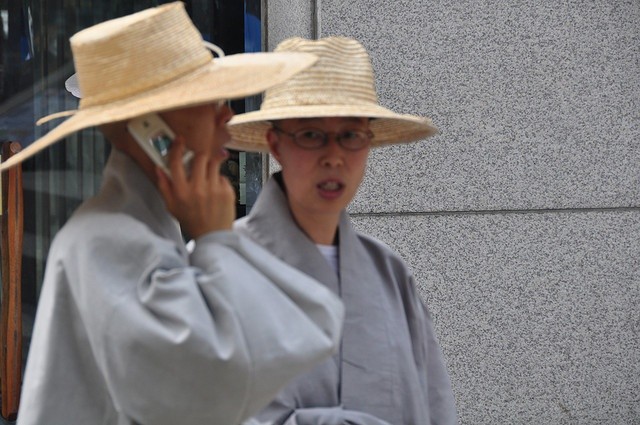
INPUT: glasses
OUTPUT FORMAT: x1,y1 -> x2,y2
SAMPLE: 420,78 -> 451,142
275,127 -> 372,150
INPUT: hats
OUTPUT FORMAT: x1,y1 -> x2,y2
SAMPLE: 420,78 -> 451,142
224,36 -> 437,153
1,1 -> 320,171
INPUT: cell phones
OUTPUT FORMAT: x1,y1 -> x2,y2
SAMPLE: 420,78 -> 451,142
126,114 -> 196,182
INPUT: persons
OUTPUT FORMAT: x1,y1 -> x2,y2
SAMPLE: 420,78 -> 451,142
185,36 -> 461,425
0,0 -> 346,425
